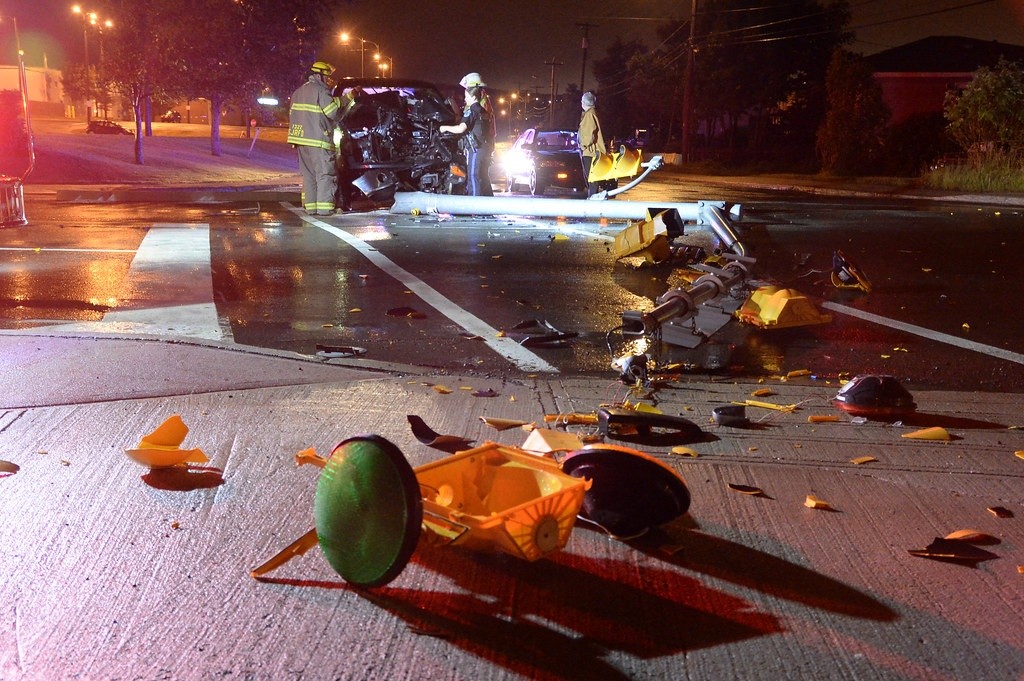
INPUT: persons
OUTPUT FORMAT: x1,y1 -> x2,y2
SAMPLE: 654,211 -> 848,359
580,92 -> 608,200
287,63 -> 360,216
439,72 -> 496,219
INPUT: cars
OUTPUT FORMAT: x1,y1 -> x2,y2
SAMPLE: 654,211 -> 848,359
502,126 -> 618,200
85,120 -> 135,137
331,76 -> 470,212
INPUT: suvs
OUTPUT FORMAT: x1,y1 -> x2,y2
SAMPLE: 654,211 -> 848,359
160,110 -> 181,123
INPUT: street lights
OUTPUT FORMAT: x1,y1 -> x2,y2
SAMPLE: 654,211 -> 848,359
340,33 -> 364,79
499,92 -> 527,148
73,5 -> 112,120
375,54 -> 392,78
360,39 -> 380,78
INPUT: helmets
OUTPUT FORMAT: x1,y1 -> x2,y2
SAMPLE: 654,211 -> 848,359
311,62 -> 333,76
459,73 -> 487,89
